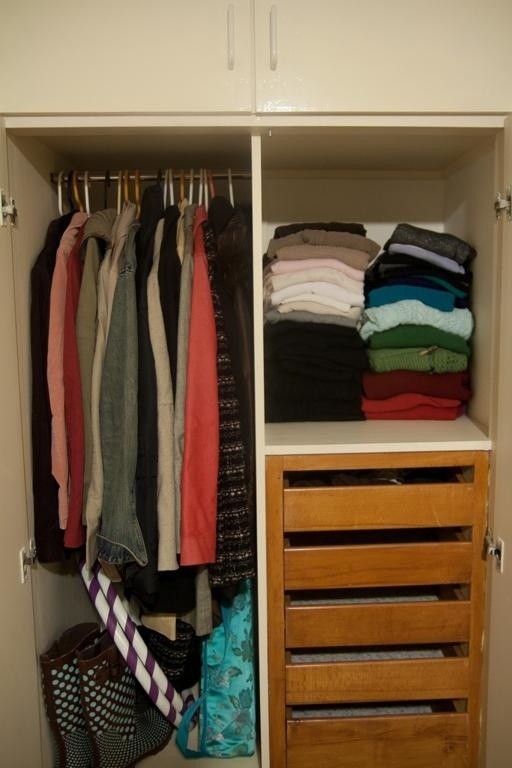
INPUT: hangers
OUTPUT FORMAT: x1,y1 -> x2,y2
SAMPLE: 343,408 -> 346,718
55,167 -> 234,216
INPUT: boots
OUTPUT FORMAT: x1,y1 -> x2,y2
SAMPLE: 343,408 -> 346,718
76,631 -> 175,767
39,621 -> 103,768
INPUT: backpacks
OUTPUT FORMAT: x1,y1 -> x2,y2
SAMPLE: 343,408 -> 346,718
176,578 -> 257,759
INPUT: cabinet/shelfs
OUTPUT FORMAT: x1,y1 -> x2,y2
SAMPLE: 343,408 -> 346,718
1,0 -> 512,767
